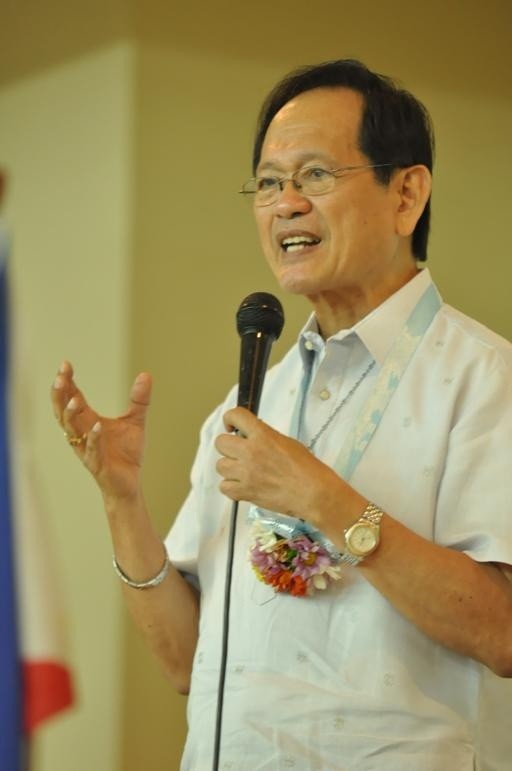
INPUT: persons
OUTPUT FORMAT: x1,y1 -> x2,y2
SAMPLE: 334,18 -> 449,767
51,59 -> 512,771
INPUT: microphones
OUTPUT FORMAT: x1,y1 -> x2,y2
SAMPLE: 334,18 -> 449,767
232,291 -> 285,437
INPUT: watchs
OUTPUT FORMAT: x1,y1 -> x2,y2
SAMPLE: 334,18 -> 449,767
335,501 -> 385,568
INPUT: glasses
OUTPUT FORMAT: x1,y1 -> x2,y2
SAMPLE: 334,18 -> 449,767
239,161 -> 413,206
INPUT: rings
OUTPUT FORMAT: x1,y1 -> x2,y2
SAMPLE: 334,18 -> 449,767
63,430 -> 88,446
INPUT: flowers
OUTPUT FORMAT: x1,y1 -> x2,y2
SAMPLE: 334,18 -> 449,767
249,527 -> 342,598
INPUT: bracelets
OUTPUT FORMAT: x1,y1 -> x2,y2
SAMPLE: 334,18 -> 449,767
111,545 -> 170,590
